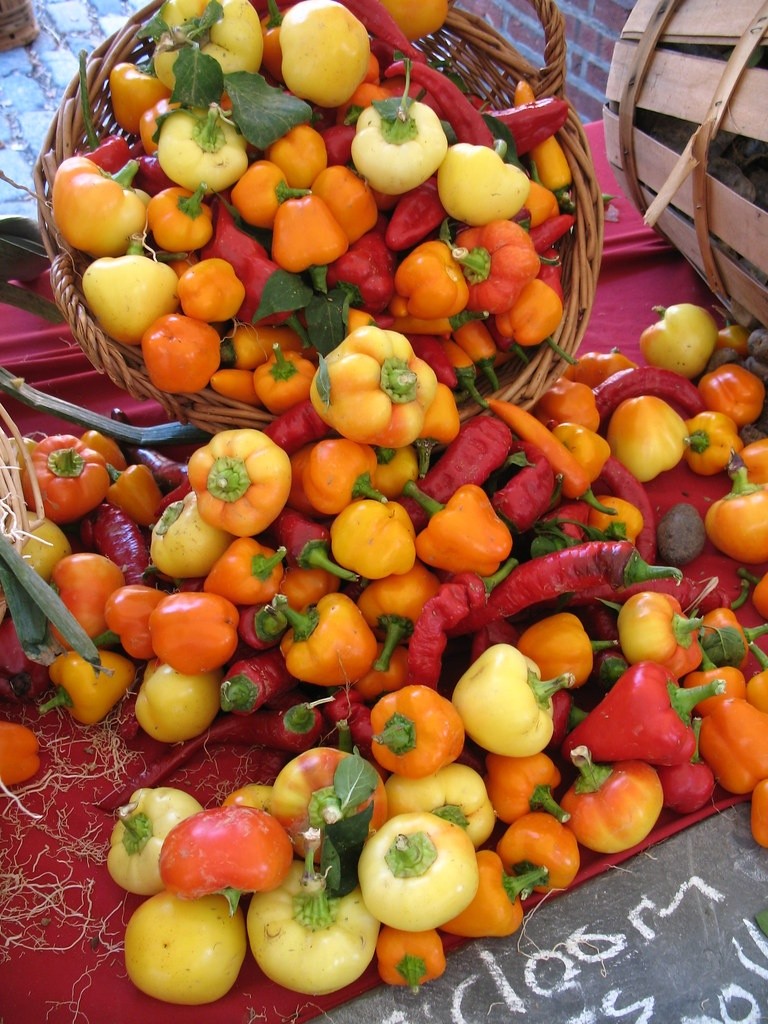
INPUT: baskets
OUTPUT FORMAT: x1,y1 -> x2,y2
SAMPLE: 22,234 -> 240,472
30,0 -> 606,454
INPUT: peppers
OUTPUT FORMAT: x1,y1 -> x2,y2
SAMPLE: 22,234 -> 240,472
0,0 -> 768,1004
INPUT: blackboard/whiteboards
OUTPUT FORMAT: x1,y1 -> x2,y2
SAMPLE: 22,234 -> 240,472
302,799 -> 768,1024
